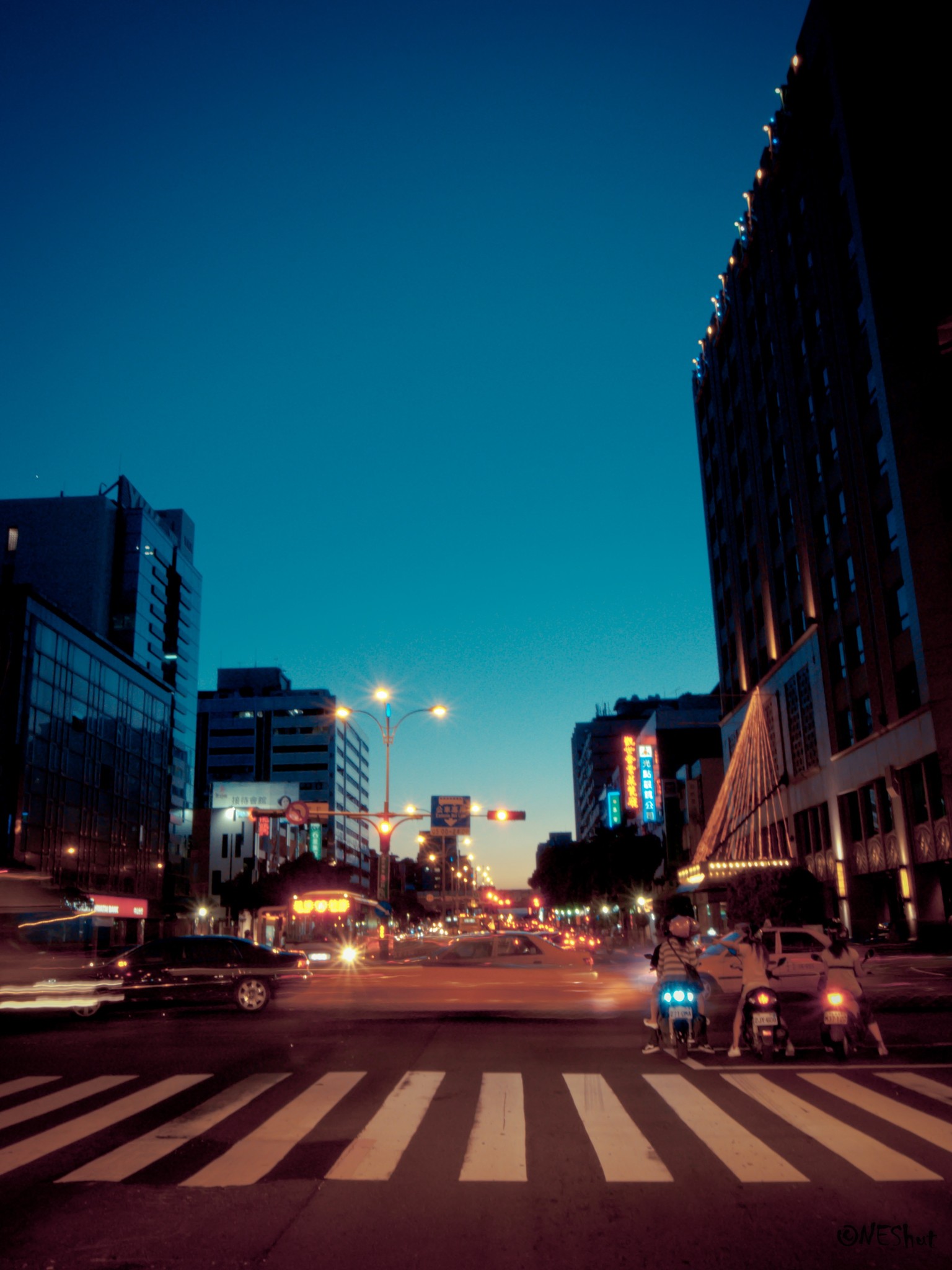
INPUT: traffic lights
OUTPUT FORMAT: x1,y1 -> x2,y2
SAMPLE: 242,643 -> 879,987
486,810 -> 527,820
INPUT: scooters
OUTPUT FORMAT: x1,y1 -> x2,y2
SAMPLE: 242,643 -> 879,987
643,948 -> 706,1060
727,949 -> 790,1063
808,948 -> 875,1062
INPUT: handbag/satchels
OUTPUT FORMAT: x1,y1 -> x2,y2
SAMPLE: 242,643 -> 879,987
684,961 -> 704,994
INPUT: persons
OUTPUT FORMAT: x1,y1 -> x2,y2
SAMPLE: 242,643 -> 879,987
820,923 -> 888,1056
711,924 -> 794,1058
641,914 -> 715,1054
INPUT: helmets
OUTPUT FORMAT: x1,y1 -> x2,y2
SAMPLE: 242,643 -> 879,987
829,923 -> 849,942
661,916 -> 671,937
670,917 -> 690,937
744,925 -> 763,940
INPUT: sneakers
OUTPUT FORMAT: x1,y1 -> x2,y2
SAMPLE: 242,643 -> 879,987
642,1043 -> 660,1055
698,1045 -> 715,1054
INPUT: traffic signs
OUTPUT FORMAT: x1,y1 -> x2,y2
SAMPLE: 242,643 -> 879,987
428,795 -> 471,837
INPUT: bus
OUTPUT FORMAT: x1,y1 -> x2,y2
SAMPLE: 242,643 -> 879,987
287,888 -> 397,966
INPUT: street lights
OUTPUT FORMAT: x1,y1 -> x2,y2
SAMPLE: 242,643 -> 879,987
331,686 -> 448,957
405,802 -> 495,933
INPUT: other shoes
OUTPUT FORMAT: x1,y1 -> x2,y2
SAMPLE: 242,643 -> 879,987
644,1018 -> 658,1029
785,1043 -> 795,1056
878,1046 -> 889,1055
727,1045 -> 741,1057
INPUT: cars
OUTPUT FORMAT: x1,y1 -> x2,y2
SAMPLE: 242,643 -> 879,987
694,928 -> 830,1001
394,929 -> 601,988
80,931 -> 314,1014
